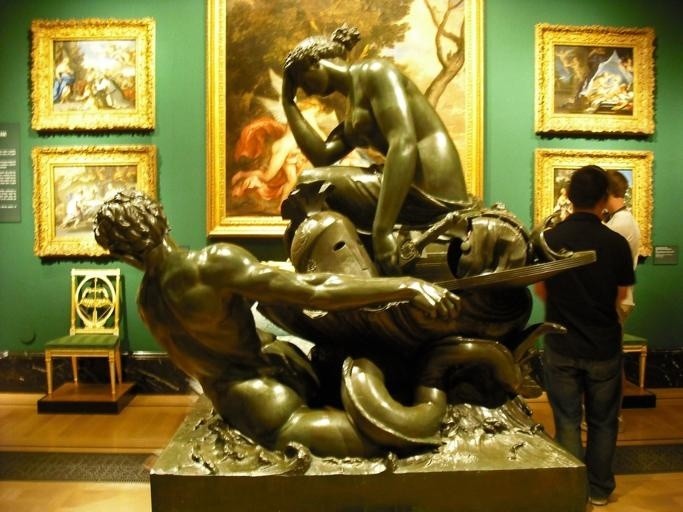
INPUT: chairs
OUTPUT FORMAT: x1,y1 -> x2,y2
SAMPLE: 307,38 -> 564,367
43,269 -> 123,397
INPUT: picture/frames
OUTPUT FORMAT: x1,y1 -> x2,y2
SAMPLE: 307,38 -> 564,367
27,15 -> 158,258
529,23 -> 656,261
202,0 -> 488,239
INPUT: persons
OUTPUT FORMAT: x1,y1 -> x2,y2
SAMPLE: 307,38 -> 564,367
556,185 -> 570,218
230,107 -> 325,184
92,190 -> 460,458
275,157 -> 300,214
281,26 -> 467,276
55,183 -> 135,231
55,46 -> 135,109
535,166 -> 636,506
579,169 -> 639,433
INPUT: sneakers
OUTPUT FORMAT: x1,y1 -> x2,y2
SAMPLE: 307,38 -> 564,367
587,496 -> 610,506
